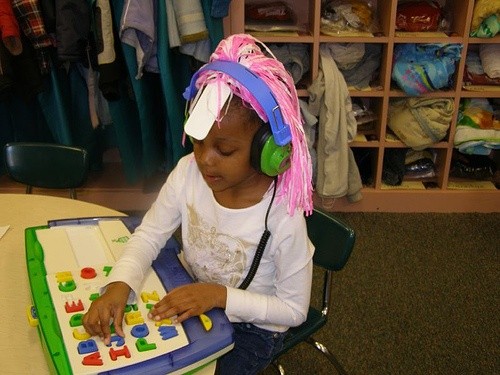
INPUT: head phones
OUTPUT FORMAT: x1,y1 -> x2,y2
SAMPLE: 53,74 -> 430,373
181,60 -> 293,178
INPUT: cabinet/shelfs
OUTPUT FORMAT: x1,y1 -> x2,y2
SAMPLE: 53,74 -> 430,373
222,0 -> 500,212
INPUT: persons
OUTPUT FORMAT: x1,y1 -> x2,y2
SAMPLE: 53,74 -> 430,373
82,33 -> 315,375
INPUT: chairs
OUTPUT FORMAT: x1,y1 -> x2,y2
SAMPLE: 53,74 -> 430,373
264,204 -> 356,375
4,143 -> 87,200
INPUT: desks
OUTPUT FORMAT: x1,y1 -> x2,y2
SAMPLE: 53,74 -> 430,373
0,193 -> 217,375
